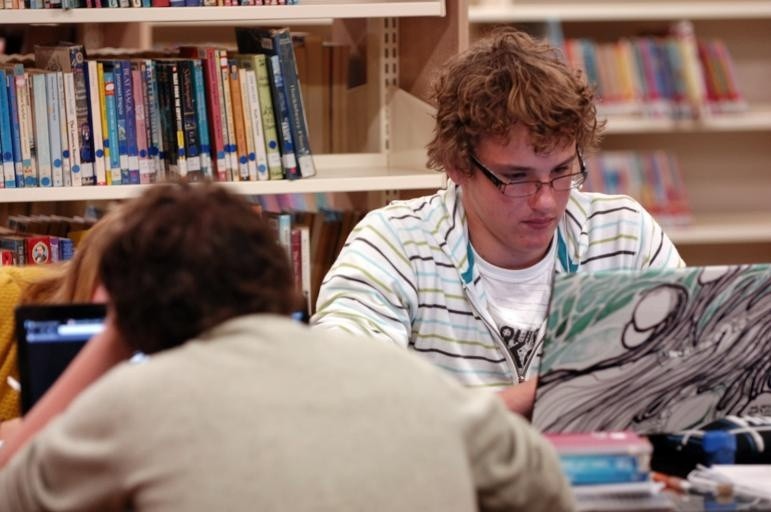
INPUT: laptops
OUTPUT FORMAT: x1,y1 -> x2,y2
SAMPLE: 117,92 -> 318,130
15,300 -> 308,422
531,262 -> 771,434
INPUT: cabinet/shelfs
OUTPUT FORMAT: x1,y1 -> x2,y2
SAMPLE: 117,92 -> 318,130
0,0 -> 771,246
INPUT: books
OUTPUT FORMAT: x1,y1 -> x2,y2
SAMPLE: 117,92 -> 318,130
1,1 -> 314,186
562,33 -> 752,235
1,202 -> 370,316
539,426 -> 771,512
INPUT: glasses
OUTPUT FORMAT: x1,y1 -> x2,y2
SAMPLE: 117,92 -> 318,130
466,142 -> 589,198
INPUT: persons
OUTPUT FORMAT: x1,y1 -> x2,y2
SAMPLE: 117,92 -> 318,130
1,206 -> 137,440
1,173 -> 577,512
306,30 -> 689,423
